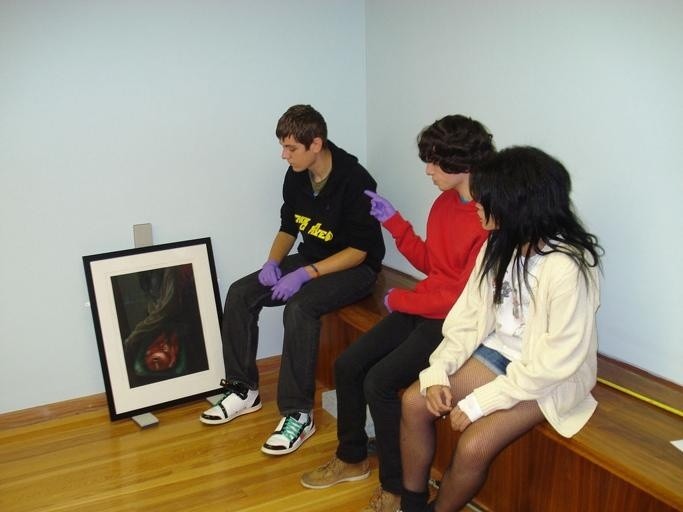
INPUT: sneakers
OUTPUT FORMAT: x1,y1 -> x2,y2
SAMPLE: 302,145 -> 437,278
199,379 -> 263,425
300,456 -> 372,490
261,409 -> 317,456
360,491 -> 402,512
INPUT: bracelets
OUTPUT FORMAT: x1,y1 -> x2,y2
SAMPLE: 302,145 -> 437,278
308,262 -> 320,277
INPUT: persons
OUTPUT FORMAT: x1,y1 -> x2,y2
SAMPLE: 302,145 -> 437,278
199,105 -> 386,459
296,114 -> 498,510
397,142 -> 604,512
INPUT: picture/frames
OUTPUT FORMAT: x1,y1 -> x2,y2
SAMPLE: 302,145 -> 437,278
83,237 -> 229,421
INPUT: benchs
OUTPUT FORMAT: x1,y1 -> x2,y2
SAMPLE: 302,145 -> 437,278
318,263 -> 683,512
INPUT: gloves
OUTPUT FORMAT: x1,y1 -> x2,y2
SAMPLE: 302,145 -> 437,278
383,288 -> 396,314
363,190 -> 398,224
257,259 -> 311,303
